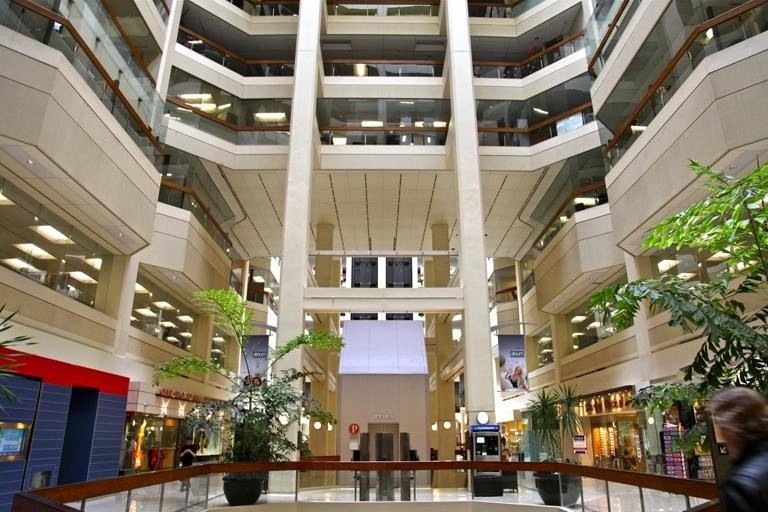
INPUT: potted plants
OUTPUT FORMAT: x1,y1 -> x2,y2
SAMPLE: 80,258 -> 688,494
151,287 -> 345,504
525,383 -> 583,505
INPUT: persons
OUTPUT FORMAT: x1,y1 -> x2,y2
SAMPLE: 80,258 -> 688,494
499,354 -> 513,391
509,366 -> 526,389
179,437 -> 197,491
502,437 -> 511,461
708,386 -> 767,511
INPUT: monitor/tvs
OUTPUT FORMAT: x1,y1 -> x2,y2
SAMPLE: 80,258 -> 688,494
473,429 -> 501,462
0,422 -> 33,461
